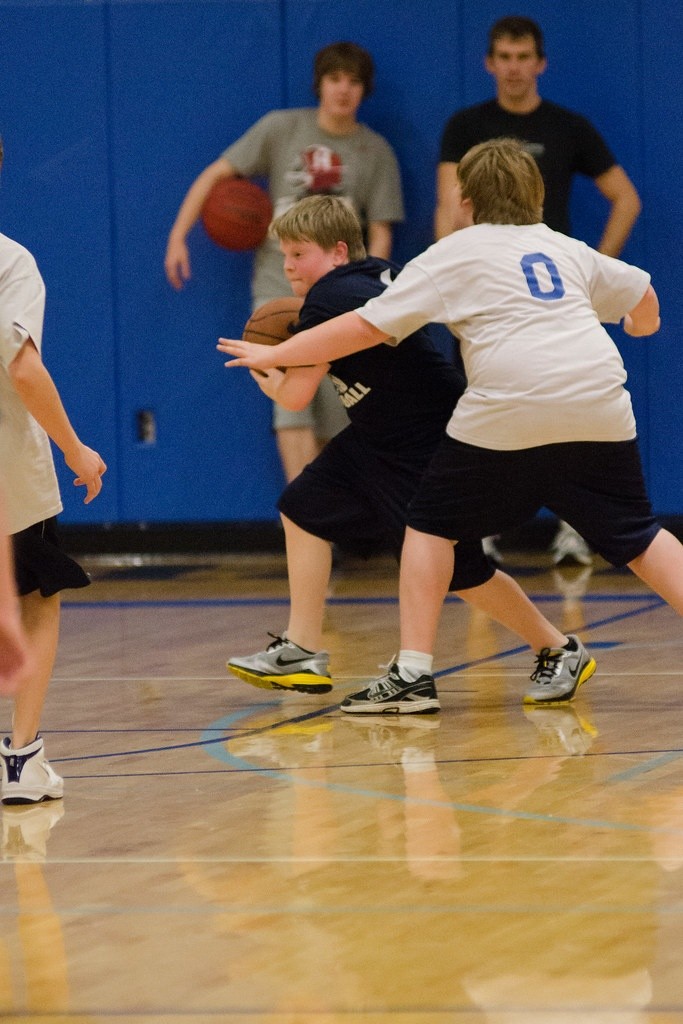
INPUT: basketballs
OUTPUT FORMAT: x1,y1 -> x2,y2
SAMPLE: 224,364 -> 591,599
241,297 -> 305,378
200,178 -> 273,250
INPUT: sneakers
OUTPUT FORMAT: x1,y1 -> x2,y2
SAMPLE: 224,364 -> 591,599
554,565 -> 593,597
524,704 -> 598,757
340,652 -> 441,713
1,799 -> 65,855
551,525 -> 590,564
225,630 -> 334,694
524,634 -> 598,705
0,731 -> 64,805
343,718 -> 442,767
226,712 -> 332,769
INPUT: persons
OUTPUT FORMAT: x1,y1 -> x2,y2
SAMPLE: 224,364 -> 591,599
435,15 -> 640,256
179,704 -> 658,1024
0,232 -> 107,805
165,42 -> 407,482
216,138 -> 683,713
2,799 -> 68,1024
226,195 -> 599,708
